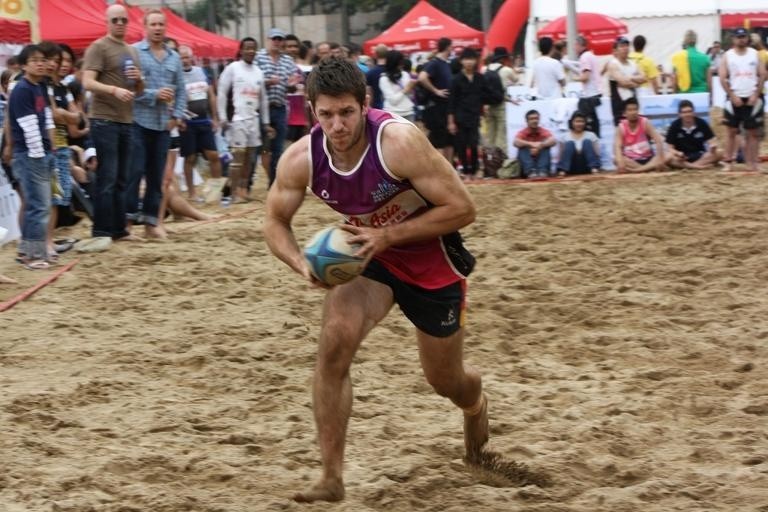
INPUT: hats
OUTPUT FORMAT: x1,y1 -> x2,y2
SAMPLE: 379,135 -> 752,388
489,45 -> 509,61
266,28 -> 286,41
732,27 -> 751,38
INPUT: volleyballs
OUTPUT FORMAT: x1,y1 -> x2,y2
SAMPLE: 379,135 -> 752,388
302,226 -> 367,286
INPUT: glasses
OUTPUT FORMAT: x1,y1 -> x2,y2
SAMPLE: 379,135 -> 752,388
109,17 -> 129,25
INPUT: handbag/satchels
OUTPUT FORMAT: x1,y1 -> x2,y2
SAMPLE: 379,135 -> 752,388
497,157 -> 523,181
482,145 -> 509,177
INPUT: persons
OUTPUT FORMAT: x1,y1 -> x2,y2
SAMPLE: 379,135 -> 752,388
253,29 -> 362,190
82,5 -> 275,243
364,37 -> 518,179
707,28 -> 768,170
514,30 -> 721,180
263,59 -> 486,502
0,41 -> 97,267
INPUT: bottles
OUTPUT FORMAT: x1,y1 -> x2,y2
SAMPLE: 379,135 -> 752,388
122,54 -> 137,88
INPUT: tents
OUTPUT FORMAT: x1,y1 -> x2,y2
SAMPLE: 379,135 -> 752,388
365,1 -> 486,58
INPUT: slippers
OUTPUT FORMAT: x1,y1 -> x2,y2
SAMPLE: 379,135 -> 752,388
13,243 -> 61,272
